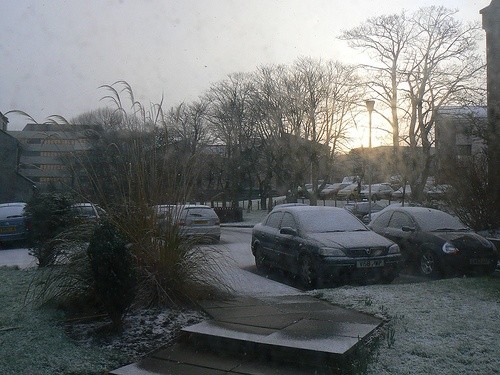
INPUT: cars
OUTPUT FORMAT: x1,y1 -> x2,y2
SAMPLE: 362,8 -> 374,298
297,181 -> 456,204
147,204 -> 175,235
68,203 -> 105,225
252,206 -> 402,291
366,206 -> 499,276
180,205 -> 222,246
1,200 -> 28,251
